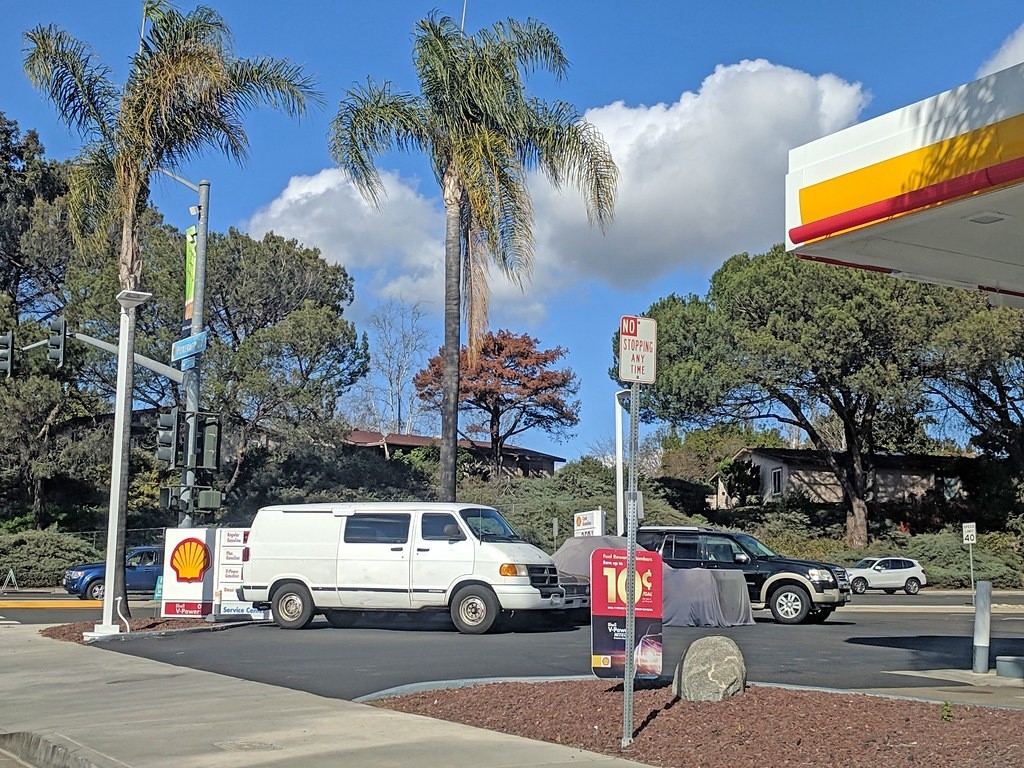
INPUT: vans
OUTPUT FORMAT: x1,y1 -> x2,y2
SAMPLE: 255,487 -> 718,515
237,501 -> 564,634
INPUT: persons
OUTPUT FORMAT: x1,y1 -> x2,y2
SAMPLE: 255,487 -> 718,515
144,556 -> 154,567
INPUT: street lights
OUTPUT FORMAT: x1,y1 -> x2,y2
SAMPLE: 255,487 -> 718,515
90,290 -> 150,638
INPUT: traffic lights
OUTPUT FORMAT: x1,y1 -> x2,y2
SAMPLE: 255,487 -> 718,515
48,315 -> 66,370
0,329 -> 13,380
156,403 -> 222,473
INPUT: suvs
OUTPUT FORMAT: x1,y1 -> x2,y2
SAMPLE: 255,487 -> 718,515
843,556 -> 927,594
634,525 -> 853,625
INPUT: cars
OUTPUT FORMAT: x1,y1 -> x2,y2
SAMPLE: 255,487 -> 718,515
508,570 -> 590,632
63,545 -> 163,601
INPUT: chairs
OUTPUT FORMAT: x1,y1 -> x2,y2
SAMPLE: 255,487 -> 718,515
707,540 -> 736,562
